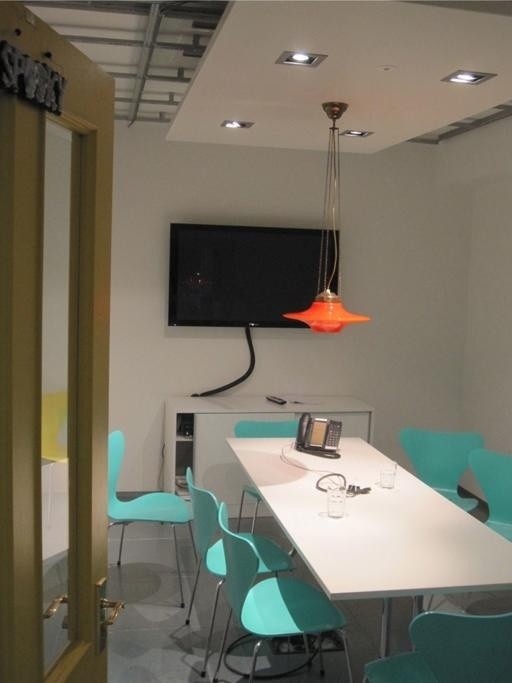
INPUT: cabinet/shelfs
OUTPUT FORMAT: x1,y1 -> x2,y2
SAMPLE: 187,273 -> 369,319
163,393 -> 374,518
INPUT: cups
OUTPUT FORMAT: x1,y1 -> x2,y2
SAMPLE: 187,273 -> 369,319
326,484 -> 345,518
379,458 -> 396,488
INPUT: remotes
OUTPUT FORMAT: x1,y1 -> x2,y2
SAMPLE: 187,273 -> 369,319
266,395 -> 287,404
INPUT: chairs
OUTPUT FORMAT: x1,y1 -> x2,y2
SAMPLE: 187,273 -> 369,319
466,447 -> 511,546
363,609 -> 512,682
184,466 -> 298,677
396,428 -> 484,515
212,501 -> 355,683
108,430 -> 200,609
235,410 -> 313,438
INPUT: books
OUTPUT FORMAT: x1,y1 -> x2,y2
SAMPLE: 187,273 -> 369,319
175,474 -> 191,500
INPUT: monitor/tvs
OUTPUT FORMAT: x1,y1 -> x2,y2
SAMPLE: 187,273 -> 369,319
168,223 -> 339,329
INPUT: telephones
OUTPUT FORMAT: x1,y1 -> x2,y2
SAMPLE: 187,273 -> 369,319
295,413 -> 341,452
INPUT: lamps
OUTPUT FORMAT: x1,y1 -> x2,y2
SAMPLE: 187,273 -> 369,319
280,102 -> 371,336
